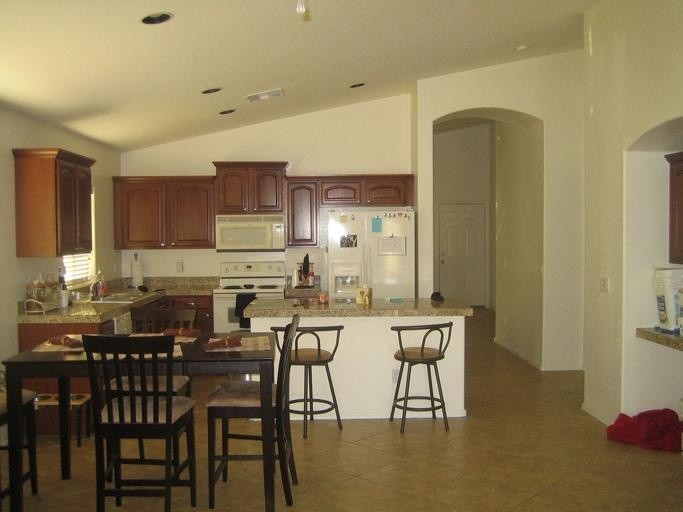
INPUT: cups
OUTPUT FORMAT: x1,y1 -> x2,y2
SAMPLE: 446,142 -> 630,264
60,289 -> 71,308
356,287 -> 371,304
317,292 -> 329,303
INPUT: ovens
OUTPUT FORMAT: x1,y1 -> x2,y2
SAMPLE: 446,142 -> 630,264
212,293 -> 284,333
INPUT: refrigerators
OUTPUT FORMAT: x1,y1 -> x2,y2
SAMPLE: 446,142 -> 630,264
330,207 -> 416,298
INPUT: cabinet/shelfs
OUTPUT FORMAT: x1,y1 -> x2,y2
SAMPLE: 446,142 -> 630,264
12,148 -> 97,258
167,297 -> 213,334
286,176 -> 320,247
212,161 -> 288,215
112,176 -> 216,249
320,174 -> 413,207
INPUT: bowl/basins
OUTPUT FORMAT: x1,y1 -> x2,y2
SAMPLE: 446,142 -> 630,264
70,291 -> 93,304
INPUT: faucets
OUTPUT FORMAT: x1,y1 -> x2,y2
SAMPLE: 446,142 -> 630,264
89,280 -> 105,300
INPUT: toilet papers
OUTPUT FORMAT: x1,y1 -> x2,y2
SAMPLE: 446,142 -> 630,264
130,253 -> 144,288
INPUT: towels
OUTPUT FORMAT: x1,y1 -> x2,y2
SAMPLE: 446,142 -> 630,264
165,327 -> 201,337
235,294 -> 257,328
50,336 -> 82,351
207,334 -> 243,350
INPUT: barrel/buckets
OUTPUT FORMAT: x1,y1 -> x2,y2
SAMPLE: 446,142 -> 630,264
650,263 -> 683,331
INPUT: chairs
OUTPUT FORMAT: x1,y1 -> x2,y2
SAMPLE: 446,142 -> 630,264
389,321 -> 453,434
271,326 -> 344,438
1,387 -> 38,512
81,335 -> 197,512
207,313 -> 300,512
103,308 -> 196,483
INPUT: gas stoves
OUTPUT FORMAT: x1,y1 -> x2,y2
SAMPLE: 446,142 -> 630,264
212,283 -> 285,292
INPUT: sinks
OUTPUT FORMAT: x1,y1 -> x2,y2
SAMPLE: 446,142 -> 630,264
91,292 -> 153,304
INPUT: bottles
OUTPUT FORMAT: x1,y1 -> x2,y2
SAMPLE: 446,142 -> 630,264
25,274 -> 59,311
291,268 -> 314,287
97,282 -> 108,297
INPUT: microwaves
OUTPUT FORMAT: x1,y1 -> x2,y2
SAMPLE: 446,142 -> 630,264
216,216 -> 285,251
